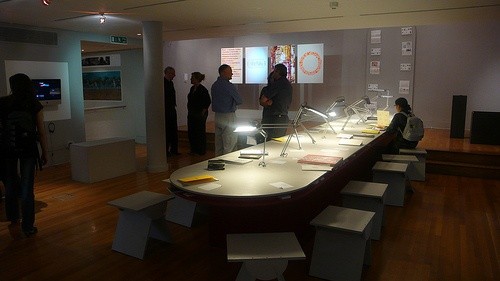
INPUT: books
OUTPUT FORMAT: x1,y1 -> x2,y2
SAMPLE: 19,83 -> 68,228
304,129 -> 320,133
337,133 -> 353,139
353,116 -> 391,137
339,139 -> 364,145
238,150 -> 268,159
298,154 -> 343,166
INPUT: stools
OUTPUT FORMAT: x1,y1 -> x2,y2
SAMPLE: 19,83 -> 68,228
165,185 -> 198,230
225,232 -> 306,281
372,161 -> 410,209
339,180 -> 390,241
399,148 -> 428,182
106,190 -> 176,260
381,154 -> 420,171
309,204 -> 377,281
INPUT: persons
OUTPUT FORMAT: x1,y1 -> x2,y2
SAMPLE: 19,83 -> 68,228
187,72 -> 211,156
257,64 -> 293,143
0,73 -> 49,236
386,98 -> 418,155
164,67 -> 181,157
211,64 -> 239,157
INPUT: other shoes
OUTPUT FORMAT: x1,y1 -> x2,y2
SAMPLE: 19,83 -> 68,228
172,151 -> 181,155
6,214 -> 20,220
166,152 -> 171,155
23,226 -> 36,235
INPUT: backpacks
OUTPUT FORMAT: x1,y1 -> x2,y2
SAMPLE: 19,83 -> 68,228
398,111 -> 424,141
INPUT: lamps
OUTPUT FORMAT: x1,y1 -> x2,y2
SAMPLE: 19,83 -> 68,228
231,95 -> 390,169
98,15 -> 106,25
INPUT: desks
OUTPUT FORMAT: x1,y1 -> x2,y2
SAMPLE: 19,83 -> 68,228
469,110 -> 500,145
168,112 -> 397,251
449,94 -> 468,139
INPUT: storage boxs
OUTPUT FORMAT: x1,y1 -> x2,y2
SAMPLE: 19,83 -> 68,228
68,135 -> 137,185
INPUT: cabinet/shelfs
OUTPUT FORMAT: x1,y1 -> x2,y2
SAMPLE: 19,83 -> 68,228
41,119 -> 71,167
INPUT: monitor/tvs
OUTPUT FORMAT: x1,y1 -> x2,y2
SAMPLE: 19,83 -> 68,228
31,79 -> 62,105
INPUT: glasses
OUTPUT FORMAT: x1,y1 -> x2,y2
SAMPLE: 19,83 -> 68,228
394,105 -> 398,107
